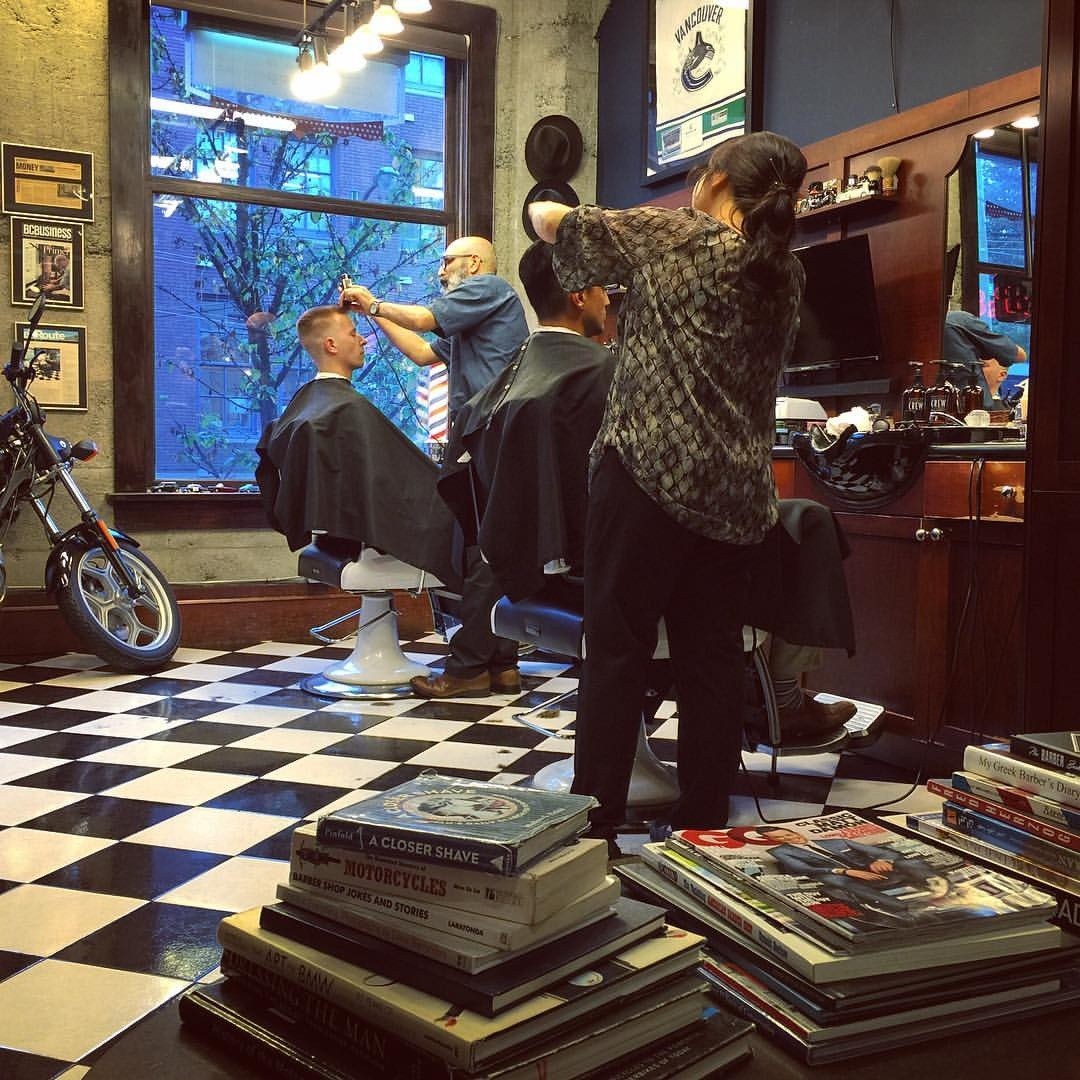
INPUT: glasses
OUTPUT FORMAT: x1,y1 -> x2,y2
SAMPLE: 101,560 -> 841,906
439,254 -> 483,268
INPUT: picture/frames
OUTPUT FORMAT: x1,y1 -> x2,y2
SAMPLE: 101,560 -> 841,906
10,216 -> 85,310
15,321 -> 88,411
1,142 -> 94,223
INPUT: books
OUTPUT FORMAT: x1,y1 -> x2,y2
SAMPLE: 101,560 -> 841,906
180,731 -> 1080,1080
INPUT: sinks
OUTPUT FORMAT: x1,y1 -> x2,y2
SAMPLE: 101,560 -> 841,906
791,430 -> 932,509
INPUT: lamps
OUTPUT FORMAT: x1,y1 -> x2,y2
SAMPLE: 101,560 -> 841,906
290,0 -> 431,99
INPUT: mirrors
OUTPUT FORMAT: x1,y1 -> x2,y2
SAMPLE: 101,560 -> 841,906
943,113 -> 1040,421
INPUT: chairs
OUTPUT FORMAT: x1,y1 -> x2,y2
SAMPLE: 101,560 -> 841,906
268,416 -> 445,686
477,399 -> 885,808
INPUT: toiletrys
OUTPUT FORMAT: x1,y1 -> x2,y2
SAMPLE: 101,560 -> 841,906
928,359 -> 957,422
960,361 -> 986,419
902,362 -> 929,420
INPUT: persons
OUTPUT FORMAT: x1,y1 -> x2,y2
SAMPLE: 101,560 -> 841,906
530,132 -> 805,857
338,236 -> 529,699
295,306 -> 537,656
757,825 -> 998,921
943,311 -> 1026,410
511,237 -> 859,743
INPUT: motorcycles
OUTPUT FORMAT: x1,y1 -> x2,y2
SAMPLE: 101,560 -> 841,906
0,279 -> 182,674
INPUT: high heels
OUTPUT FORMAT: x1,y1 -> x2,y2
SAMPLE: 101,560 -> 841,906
743,679 -> 859,751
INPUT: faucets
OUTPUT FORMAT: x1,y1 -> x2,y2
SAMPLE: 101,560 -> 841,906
930,411 -> 966,426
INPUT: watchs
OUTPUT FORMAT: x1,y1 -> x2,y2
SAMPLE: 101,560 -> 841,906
370,300 -> 383,317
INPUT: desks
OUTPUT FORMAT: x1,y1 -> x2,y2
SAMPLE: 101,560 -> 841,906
82,1001 -> 1080,1080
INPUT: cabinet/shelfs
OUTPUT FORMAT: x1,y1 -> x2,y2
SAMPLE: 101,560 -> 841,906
770,441 -> 1026,779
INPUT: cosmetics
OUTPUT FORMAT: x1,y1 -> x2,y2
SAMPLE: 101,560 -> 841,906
864,157 -> 900,191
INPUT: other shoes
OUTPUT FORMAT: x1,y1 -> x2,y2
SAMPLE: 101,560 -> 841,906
630,800 -> 728,826
583,806 -> 625,826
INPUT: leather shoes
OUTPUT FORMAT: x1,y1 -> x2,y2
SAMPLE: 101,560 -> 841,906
490,668 -> 521,695
410,668 -> 492,698
